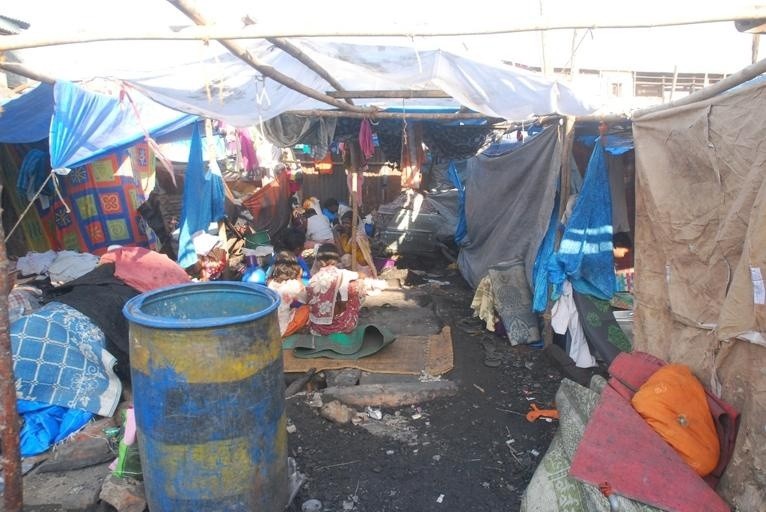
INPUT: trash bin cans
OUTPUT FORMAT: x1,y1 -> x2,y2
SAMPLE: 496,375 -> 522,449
123,281 -> 289,512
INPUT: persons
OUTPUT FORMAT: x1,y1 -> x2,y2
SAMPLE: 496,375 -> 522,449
263,195 -> 373,340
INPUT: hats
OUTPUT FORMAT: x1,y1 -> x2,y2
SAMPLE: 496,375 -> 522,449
317,244 -> 340,259
